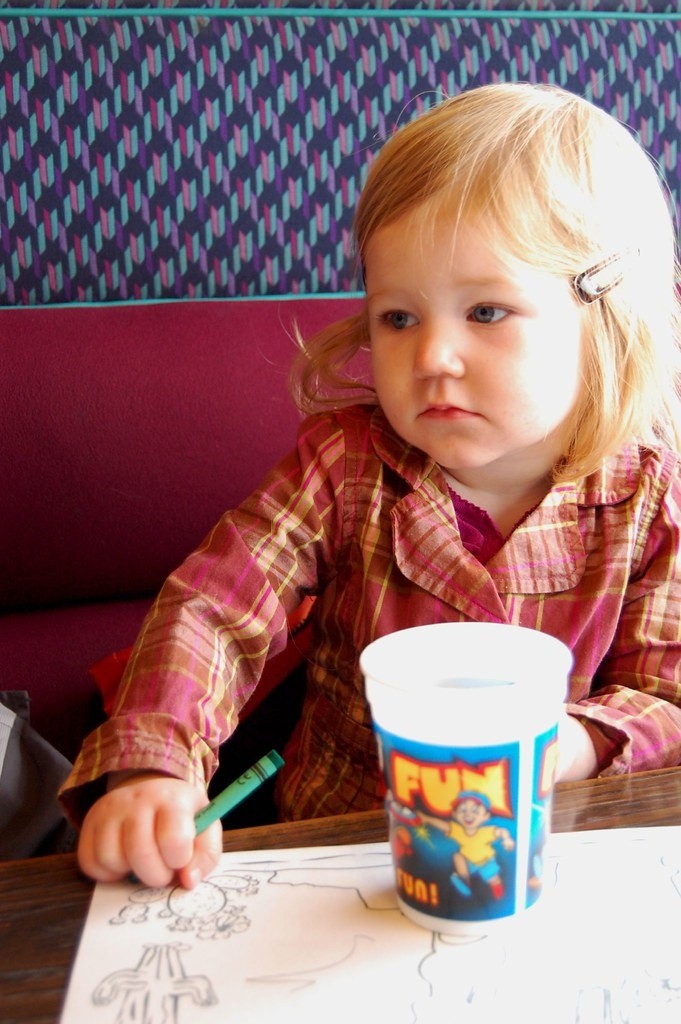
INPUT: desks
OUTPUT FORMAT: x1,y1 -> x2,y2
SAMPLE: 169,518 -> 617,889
0,763 -> 681,1024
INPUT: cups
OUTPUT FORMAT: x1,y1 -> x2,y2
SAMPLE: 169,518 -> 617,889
359,622 -> 574,933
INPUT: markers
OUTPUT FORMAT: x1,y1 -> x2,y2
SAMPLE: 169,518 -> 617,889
190,744 -> 288,839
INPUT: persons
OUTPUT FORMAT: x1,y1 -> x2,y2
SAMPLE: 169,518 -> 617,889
55,83 -> 681,891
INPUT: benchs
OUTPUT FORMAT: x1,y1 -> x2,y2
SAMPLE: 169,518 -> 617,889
0,291 -> 377,769
